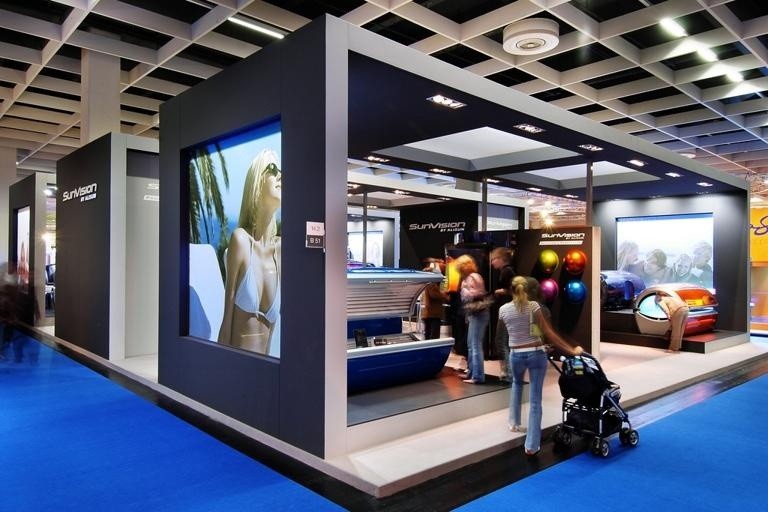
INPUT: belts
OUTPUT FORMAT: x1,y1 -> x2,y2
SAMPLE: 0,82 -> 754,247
510,346 -> 544,352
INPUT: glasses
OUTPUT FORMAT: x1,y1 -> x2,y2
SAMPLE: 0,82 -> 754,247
261,163 -> 281,176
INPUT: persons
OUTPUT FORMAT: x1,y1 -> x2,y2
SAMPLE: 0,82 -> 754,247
0,259 -> 42,365
617,240 -> 644,278
659,252 -> 698,287
440,246 -> 468,356
640,247 -> 669,288
211,144 -> 281,355
692,240 -> 714,288
420,256 -> 450,341
490,246 -> 524,388
494,273 -> 584,454
653,289 -> 688,356
15,242 -> 29,285
453,253 -> 488,385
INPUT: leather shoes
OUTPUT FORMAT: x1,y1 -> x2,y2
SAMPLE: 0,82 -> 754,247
459,374 -> 474,383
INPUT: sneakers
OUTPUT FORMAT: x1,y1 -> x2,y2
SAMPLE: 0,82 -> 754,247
509,424 -> 517,431
525,446 -> 540,455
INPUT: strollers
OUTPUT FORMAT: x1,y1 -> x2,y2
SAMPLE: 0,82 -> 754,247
537,342 -> 640,459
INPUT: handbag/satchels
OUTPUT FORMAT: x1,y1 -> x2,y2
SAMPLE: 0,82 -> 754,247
530,323 -> 543,336
464,295 -> 494,314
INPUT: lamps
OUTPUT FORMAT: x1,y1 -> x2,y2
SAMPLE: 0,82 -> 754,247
501,18 -> 558,57
